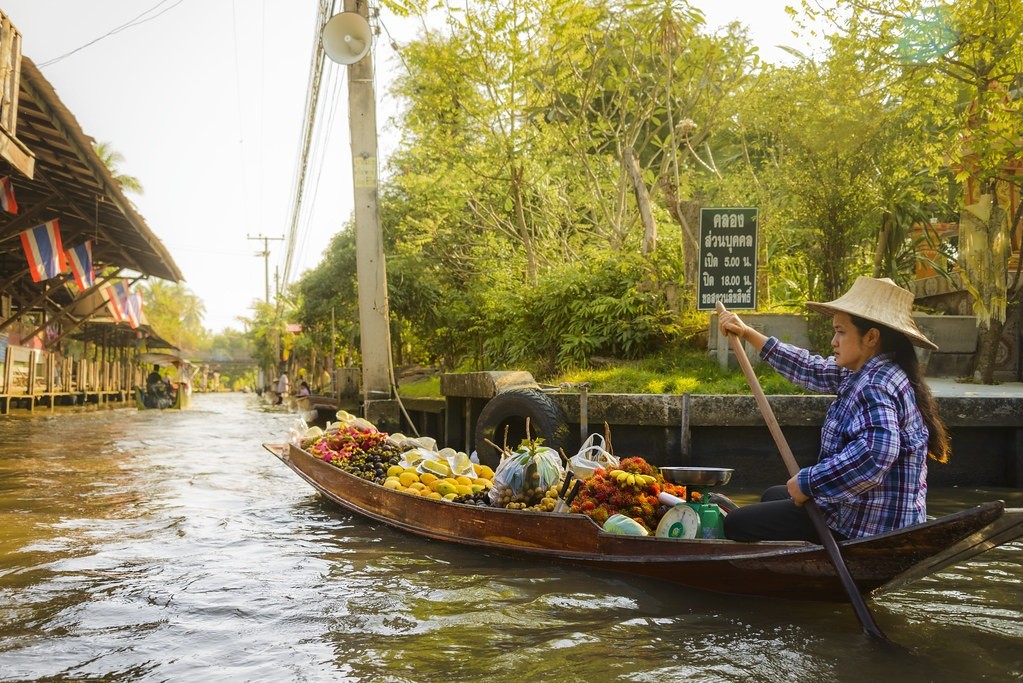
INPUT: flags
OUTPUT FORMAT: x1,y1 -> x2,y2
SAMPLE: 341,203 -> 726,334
0,176 -> 18,215
66,241 -> 95,292
135,313 -> 150,338
128,292 -> 144,329
105,278 -> 129,323
20,219 -> 67,283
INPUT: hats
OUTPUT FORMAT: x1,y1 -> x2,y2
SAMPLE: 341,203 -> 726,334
804,274 -> 940,353
272,377 -> 279,383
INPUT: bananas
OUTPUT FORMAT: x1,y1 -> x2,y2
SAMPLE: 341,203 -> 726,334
609,470 -> 657,489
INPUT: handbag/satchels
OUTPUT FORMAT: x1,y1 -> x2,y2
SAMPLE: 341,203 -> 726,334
567,432 -> 619,480
489,441 -> 566,507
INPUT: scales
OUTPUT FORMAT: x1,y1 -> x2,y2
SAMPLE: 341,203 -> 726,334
654,466 -> 734,539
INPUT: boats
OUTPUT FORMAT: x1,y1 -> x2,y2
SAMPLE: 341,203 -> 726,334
134,383 -> 190,410
263,417 -> 1023,598
296,395 -> 338,413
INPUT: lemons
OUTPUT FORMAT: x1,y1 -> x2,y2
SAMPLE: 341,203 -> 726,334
495,447 -> 576,511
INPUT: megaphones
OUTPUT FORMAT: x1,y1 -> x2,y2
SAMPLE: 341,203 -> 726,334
321,11 -> 373,65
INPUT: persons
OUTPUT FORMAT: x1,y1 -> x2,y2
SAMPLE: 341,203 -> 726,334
300,382 -> 311,395
164,372 -> 172,398
318,366 -> 331,391
718,278 -> 950,544
298,364 -> 307,381
278,371 -> 293,402
147,365 -> 167,397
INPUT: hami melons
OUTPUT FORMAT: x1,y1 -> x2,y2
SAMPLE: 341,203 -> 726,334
384,448 -> 494,500
